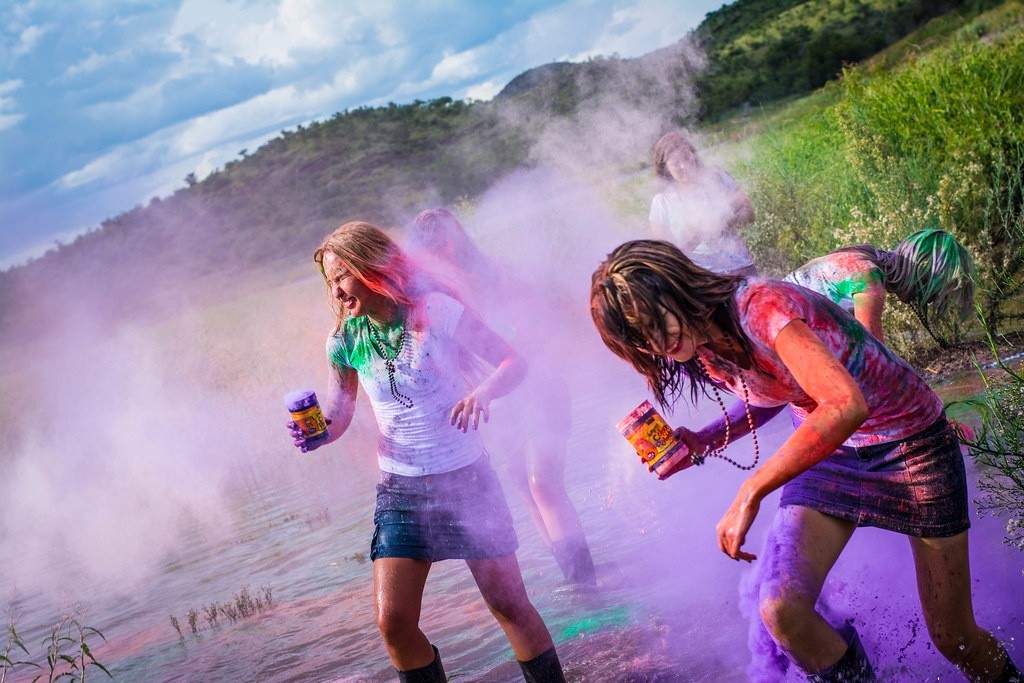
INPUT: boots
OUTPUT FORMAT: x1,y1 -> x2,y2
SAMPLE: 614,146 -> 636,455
805,626 -> 875,683
395,645 -> 447,683
966,650 -> 1024,683
516,646 -> 567,683
550,532 -> 595,584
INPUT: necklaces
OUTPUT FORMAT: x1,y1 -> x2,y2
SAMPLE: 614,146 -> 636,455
365,311 -> 413,408
691,334 -> 759,470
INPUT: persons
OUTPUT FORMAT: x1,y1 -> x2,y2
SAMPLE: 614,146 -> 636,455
590,239 -> 1024,683
650,129 -> 763,277
406,208 -> 597,587
781,229 -> 975,344
286,221 -> 566,683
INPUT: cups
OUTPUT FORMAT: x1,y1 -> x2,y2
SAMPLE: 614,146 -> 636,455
616,399 -> 690,480
284,387 -> 332,446
